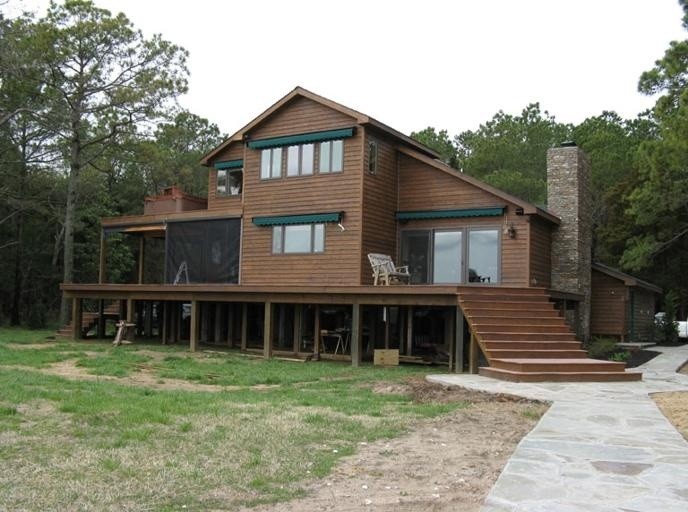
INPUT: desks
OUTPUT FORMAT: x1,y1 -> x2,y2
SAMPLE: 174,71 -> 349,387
319,334 -> 345,355
336,328 -> 376,355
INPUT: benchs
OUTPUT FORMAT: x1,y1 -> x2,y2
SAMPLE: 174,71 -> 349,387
591,330 -> 631,342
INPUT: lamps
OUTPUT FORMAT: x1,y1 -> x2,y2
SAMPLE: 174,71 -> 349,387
508,222 -> 516,238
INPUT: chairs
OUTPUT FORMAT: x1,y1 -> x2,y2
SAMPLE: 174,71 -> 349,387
469,268 -> 491,282
367,253 -> 411,286
